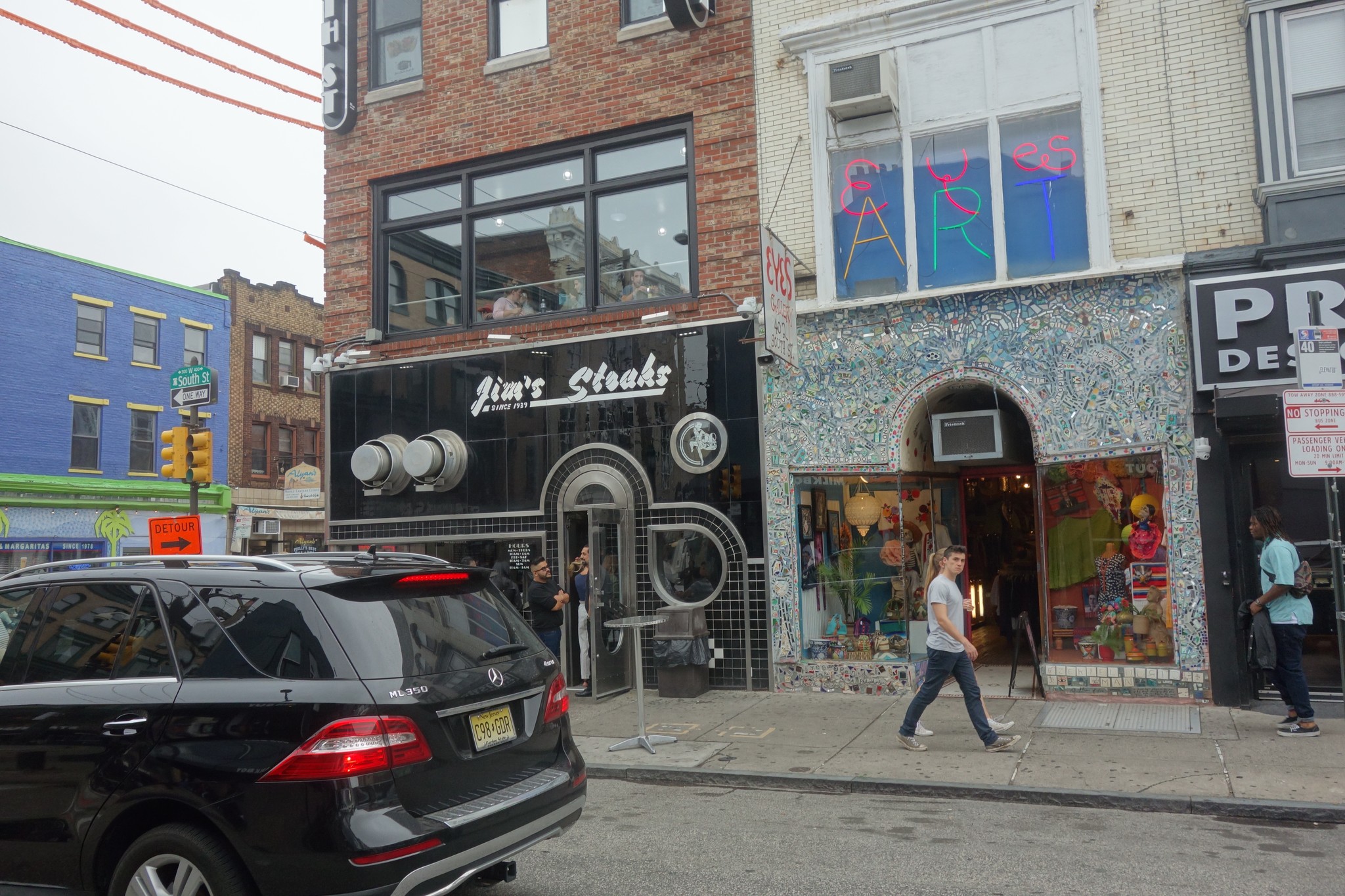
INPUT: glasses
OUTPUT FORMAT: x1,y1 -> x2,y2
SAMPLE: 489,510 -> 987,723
532,564 -> 550,573
513,284 -> 518,287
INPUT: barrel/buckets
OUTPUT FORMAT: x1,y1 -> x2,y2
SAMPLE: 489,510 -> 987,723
830,645 -> 848,660
809,639 -> 831,660
1053,605 -> 1078,628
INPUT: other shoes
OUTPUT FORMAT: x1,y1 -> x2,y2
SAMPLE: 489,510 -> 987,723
583,681 -> 588,686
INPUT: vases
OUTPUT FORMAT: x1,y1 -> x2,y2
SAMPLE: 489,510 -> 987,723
1078,636 -> 1097,659
1133,615 -> 1151,634
1053,605 -> 1078,629
809,639 -> 847,660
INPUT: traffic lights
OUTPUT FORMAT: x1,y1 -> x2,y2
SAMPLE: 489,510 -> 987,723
161,427 -> 188,479
185,430 -> 213,483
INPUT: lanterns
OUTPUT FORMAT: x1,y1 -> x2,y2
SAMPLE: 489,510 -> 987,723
1121,523 -> 1133,543
1130,494 -> 1159,519
844,476 -> 881,537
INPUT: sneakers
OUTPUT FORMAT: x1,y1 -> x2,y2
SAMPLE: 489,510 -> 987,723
984,734 -> 1021,752
1276,716 -> 1298,728
1276,723 -> 1321,738
987,718 -> 1014,734
896,731 -> 928,750
914,720 -> 935,735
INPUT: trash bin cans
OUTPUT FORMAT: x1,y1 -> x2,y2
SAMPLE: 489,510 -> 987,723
651,605 -> 708,699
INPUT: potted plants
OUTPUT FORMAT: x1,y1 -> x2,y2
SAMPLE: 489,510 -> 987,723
815,533 -> 887,634
879,598 -> 908,632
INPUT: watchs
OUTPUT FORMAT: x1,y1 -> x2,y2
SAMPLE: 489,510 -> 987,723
1255,599 -> 1262,606
587,615 -> 590,620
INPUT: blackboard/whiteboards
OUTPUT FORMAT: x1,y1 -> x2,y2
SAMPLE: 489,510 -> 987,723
1011,611 -> 1043,689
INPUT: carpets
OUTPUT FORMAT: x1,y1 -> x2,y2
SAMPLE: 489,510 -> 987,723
936,664 -> 1045,700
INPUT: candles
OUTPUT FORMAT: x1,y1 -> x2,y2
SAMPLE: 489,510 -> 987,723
1090,618 -> 1125,662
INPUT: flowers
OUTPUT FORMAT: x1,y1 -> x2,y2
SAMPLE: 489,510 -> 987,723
879,538 -> 911,566
913,586 -> 925,599
1100,597 -> 1166,624
1045,457 -> 1147,484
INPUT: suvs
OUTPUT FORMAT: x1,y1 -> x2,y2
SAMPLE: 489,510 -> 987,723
1,544 -> 590,896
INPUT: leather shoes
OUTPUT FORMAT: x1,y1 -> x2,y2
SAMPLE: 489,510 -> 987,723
575,688 -> 592,697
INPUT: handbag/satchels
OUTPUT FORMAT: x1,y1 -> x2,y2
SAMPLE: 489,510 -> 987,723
1287,561 -> 1315,600
854,617 -> 871,636
888,635 -> 907,653
826,613 -> 847,635
872,630 -> 889,650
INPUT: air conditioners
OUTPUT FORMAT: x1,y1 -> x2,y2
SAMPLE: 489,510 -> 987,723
278,375 -> 300,388
824,49 -> 899,120
252,521 -> 280,535
932,409 -> 1013,461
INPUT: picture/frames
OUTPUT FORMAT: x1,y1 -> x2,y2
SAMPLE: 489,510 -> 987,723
912,599 -> 928,621
798,488 -> 840,558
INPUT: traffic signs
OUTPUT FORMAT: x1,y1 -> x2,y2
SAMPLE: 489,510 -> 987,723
170,382 -> 211,409
148,514 -> 204,556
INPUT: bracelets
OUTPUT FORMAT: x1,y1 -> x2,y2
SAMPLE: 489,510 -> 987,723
561,595 -> 563,602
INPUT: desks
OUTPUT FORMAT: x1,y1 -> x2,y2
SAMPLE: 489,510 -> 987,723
603,615 -> 677,754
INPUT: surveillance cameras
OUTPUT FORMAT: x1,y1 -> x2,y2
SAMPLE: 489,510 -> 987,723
736,304 -> 754,319
310,362 -> 323,377
333,355 -> 350,369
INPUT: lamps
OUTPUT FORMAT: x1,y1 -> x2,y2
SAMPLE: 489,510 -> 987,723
487,334 -> 527,343
641,310 -> 677,323
349,350 -> 388,359
844,476 -> 880,536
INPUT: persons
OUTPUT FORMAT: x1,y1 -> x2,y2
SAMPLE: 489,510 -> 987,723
896,546 -> 1022,753
802,551 -> 815,575
481,269 -> 688,322
1094,543 -> 1129,622
527,556 -> 572,658
900,529 -> 922,601
567,544 -> 619,697
1248,506 -> 1320,737
1141,585 -> 1169,648
461,557 -> 491,641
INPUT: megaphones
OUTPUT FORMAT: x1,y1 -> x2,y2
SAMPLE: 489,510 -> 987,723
757,351 -> 775,367
